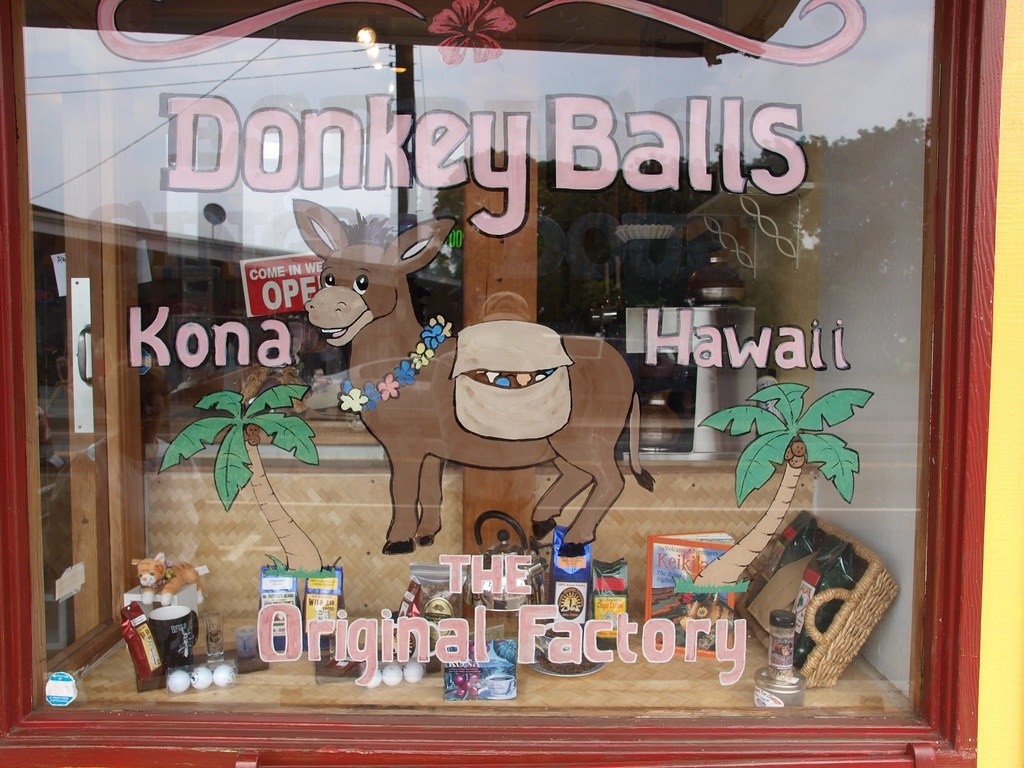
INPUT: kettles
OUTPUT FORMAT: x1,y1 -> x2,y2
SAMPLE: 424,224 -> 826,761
464,512 -> 548,609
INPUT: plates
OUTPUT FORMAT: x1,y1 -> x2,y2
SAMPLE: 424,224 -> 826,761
527,649 -> 605,677
478,688 -> 516,700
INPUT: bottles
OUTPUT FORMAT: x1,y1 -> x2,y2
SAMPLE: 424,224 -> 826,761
767,610 -> 796,679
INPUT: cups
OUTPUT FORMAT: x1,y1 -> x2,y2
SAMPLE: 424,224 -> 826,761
204,611 -> 224,657
485,675 -> 515,694
235,627 -> 256,659
754,668 -> 805,708
535,636 -> 570,662
149,606 -> 199,669
